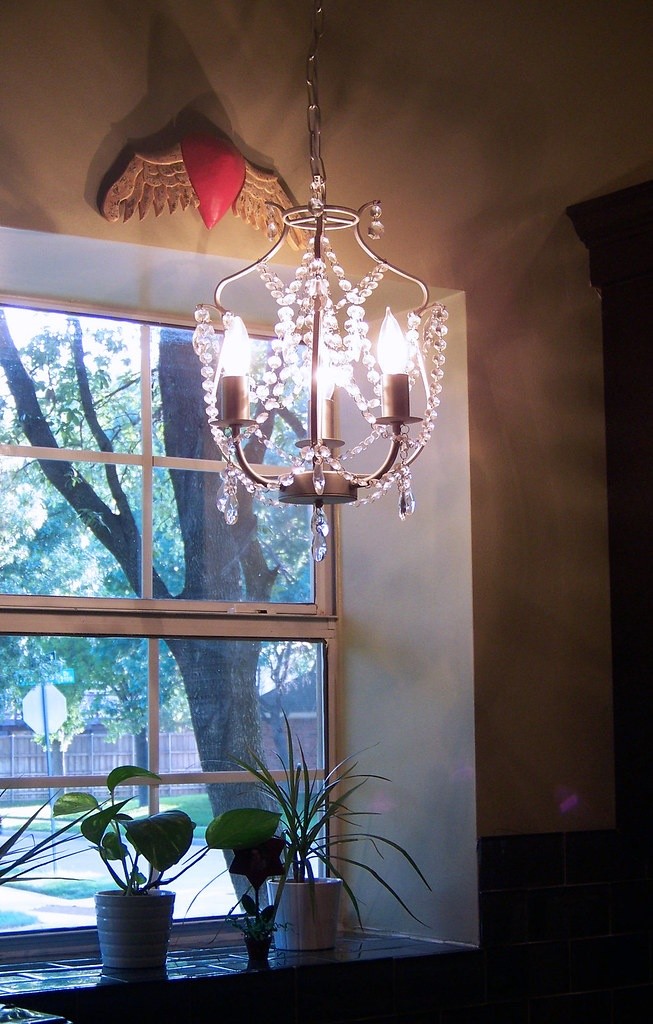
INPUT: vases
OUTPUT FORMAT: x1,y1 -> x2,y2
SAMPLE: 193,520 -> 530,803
243,937 -> 271,970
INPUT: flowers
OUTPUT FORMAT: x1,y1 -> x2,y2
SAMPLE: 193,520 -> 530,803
224,837 -> 291,940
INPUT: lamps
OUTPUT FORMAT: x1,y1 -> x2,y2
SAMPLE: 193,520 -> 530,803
192,2 -> 451,564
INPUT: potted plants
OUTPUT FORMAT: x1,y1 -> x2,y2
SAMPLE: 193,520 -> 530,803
50,764 -> 284,971
180,699 -> 433,949
